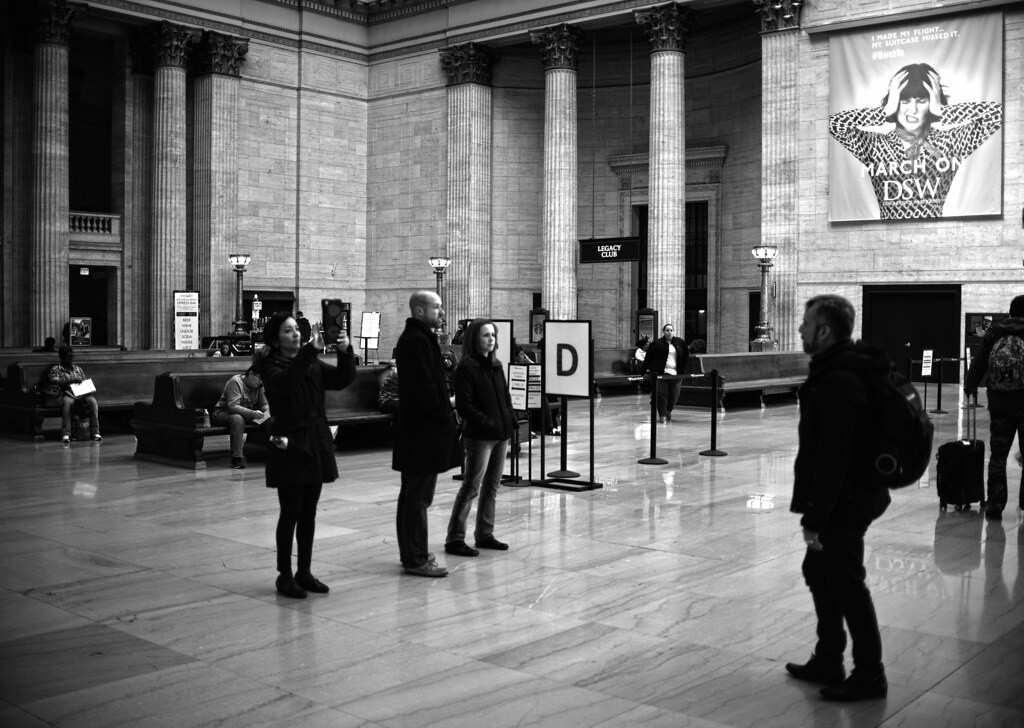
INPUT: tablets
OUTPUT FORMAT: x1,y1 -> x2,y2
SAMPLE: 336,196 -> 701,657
322,299 -> 344,345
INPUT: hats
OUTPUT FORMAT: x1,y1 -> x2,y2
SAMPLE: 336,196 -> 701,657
443,350 -> 454,362
59,346 -> 72,359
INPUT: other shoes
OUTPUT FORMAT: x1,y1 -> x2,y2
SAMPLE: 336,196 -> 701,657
786,654 -> 888,700
279,570 -> 328,598
446,536 -> 508,556
660,411 -> 671,423
986,502 -> 1002,519
405,552 -> 448,577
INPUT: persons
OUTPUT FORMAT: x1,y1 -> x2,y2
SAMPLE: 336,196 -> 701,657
442,351 -> 465,444
964,294 -> 1024,522
644,324 -> 690,424
254,311 -> 358,599
828,62 -> 1003,220
296,310 -> 312,349
634,336 -> 650,376
452,318 -> 520,557
48,346 -> 104,443
212,342 -> 237,357
212,364 -> 271,469
450,320 -> 467,345
378,362 -> 402,414
390,289 -> 466,579
785,295 -> 895,703
33,337 -> 61,353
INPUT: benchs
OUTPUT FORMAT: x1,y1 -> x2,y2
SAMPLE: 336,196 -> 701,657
592,348 -> 649,398
677,351 -> 810,413
0,345 -> 561,470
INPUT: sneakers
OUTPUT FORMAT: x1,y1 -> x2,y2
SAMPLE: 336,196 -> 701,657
91,433 -> 103,440
62,434 -> 71,443
231,457 -> 245,469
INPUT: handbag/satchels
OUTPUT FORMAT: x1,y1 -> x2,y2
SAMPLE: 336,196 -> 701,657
41,381 -> 64,408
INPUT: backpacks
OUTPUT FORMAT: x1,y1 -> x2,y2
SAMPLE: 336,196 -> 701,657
840,343 -> 930,488
985,325 -> 1024,392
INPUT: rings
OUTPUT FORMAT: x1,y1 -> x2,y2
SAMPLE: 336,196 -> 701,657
806,540 -> 814,544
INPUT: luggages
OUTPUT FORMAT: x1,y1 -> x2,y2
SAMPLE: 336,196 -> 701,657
937,389 -> 986,512
